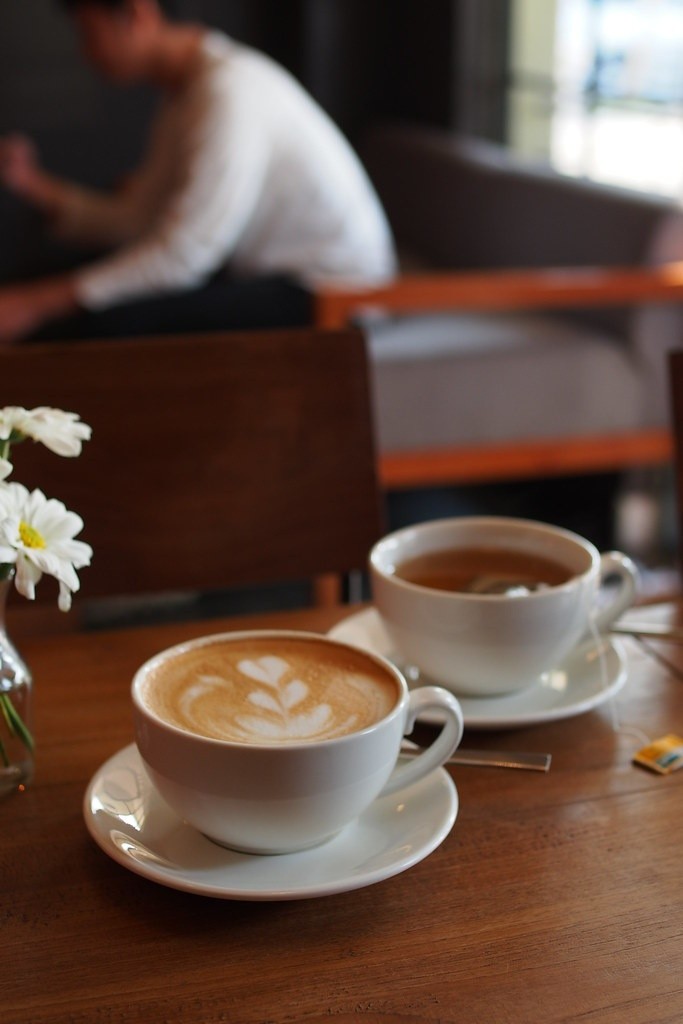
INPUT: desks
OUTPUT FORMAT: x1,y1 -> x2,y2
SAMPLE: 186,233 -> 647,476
0,567 -> 683,1024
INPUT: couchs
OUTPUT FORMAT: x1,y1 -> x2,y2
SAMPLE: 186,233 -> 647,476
312,122 -> 683,598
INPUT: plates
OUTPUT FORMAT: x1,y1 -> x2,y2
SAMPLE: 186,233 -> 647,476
325,608 -> 628,728
82,742 -> 458,901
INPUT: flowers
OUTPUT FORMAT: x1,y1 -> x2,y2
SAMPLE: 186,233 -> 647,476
0,403 -> 94,761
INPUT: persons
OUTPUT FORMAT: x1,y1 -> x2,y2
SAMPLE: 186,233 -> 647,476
0,0 -> 397,335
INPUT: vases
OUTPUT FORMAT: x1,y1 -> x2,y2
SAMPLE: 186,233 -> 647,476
1,566 -> 35,794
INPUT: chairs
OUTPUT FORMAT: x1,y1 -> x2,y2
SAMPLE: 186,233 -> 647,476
0,323 -> 382,626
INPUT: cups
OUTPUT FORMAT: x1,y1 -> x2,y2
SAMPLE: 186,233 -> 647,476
369,514 -> 638,700
129,629 -> 465,856
